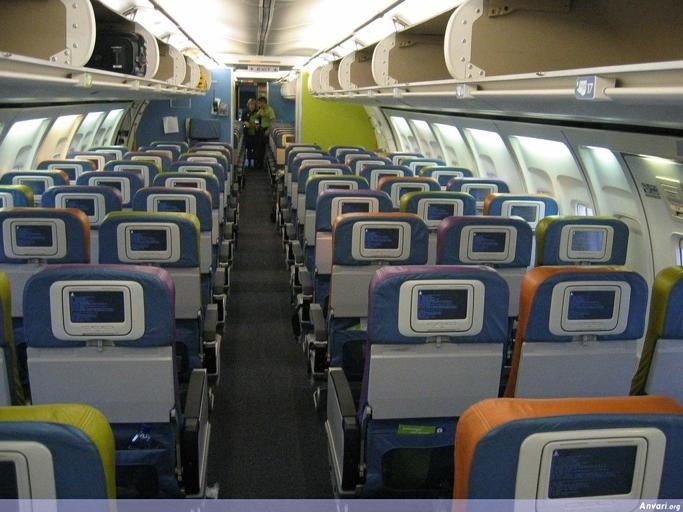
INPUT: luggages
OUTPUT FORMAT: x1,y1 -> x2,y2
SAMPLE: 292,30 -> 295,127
86,28 -> 147,77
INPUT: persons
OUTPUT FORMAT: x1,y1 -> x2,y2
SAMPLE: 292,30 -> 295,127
242,98 -> 260,168
255,96 -> 275,169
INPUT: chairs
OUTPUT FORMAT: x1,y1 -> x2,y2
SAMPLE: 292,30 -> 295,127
264,123 -> 683,499
0,120 -> 246,498
